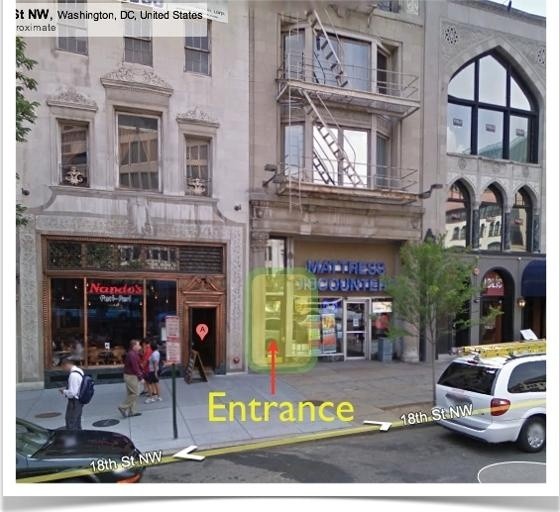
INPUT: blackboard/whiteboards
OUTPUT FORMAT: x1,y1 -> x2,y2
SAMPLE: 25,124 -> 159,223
185,350 -> 195,383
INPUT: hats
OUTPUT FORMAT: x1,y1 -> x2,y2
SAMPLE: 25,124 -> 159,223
57,355 -> 72,365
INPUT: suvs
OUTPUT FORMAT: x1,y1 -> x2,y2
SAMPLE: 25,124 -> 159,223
434,340 -> 546,453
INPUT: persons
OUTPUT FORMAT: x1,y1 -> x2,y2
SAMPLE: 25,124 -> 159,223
118,337 -> 163,417
66,337 -> 84,361
58,357 -> 96,430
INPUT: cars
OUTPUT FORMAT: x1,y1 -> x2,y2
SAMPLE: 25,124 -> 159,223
16,419 -> 145,484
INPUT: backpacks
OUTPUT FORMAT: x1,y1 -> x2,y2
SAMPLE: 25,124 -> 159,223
73,370 -> 95,403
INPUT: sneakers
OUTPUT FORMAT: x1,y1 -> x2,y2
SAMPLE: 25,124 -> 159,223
119,407 -> 129,417
140,391 -> 163,405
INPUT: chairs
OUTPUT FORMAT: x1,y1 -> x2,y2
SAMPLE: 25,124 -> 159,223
63,341 -> 125,366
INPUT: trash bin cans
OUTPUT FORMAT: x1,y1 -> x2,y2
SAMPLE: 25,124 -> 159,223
377,337 -> 393,362
